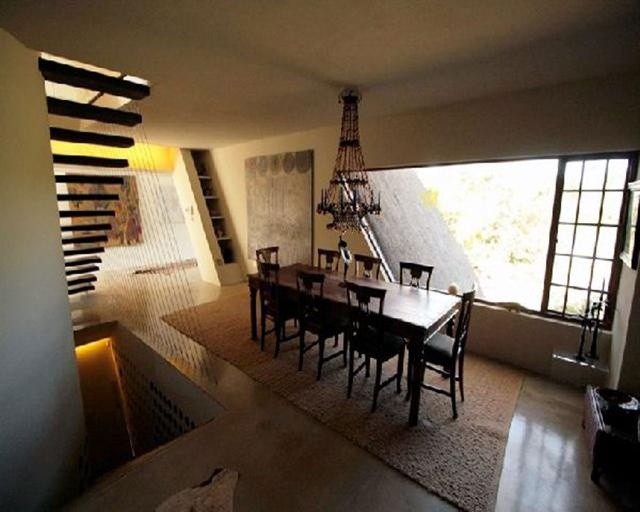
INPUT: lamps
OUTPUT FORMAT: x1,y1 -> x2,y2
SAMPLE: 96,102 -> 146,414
316,88 -> 381,264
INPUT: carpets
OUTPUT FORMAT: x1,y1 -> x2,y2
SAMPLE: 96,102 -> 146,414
159,290 -> 528,512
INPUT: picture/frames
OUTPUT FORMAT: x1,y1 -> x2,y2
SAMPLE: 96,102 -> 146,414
619,180 -> 640,269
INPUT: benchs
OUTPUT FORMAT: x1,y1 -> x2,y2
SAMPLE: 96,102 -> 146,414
582,385 -> 640,481
